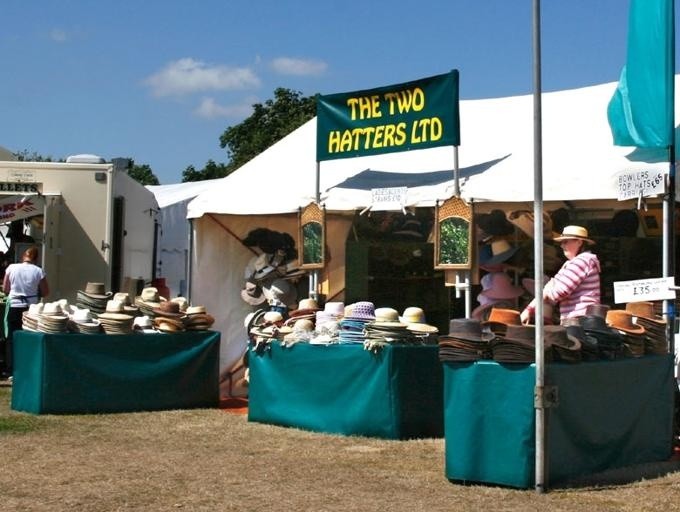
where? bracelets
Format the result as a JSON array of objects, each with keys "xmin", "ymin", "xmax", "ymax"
[{"xmin": 526, "ymin": 306, "xmax": 535, "ymax": 312}]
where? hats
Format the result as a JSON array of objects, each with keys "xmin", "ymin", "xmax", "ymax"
[
  {"xmin": 438, "ymin": 208, "xmax": 560, "ymax": 302},
  {"xmin": 553, "ymin": 225, "xmax": 597, "ymax": 245},
  {"xmin": 439, "ymin": 302, "xmax": 670, "ymax": 362},
  {"xmin": 22, "ymin": 280, "xmax": 217, "ymax": 337},
  {"xmin": 238, "ymin": 248, "xmax": 438, "ymax": 351}
]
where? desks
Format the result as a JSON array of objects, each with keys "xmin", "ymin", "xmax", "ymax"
[
  {"xmin": 244, "ymin": 331, "xmax": 444, "ymax": 440},
  {"xmin": 437, "ymin": 349, "xmax": 675, "ymax": 494},
  {"xmin": 9, "ymin": 328, "xmax": 223, "ymax": 414}
]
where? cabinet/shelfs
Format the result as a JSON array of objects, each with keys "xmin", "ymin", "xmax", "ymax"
[{"xmin": 341, "ymin": 238, "xmax": 451, "ymax": 337}]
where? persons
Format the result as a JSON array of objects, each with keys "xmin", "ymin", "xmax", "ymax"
[
  {"xmin": 0, "ymin": 247, "xmax": 48, "ymax": 377},
  {"xmin": 520, "ymin": 226, "xmax": 601, "ymax": 326}
]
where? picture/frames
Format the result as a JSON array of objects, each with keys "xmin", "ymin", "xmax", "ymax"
[
  {"xmin": 634, "ymin": 204, "xmax": 680, "ymax": 238},
  {"xmin": 430, "ymin": 193, "xmax": 475, "ymax": 271},
  {"xmin": 295, "ymin": 201, "xmax": 330, "ymax": 272}
]
